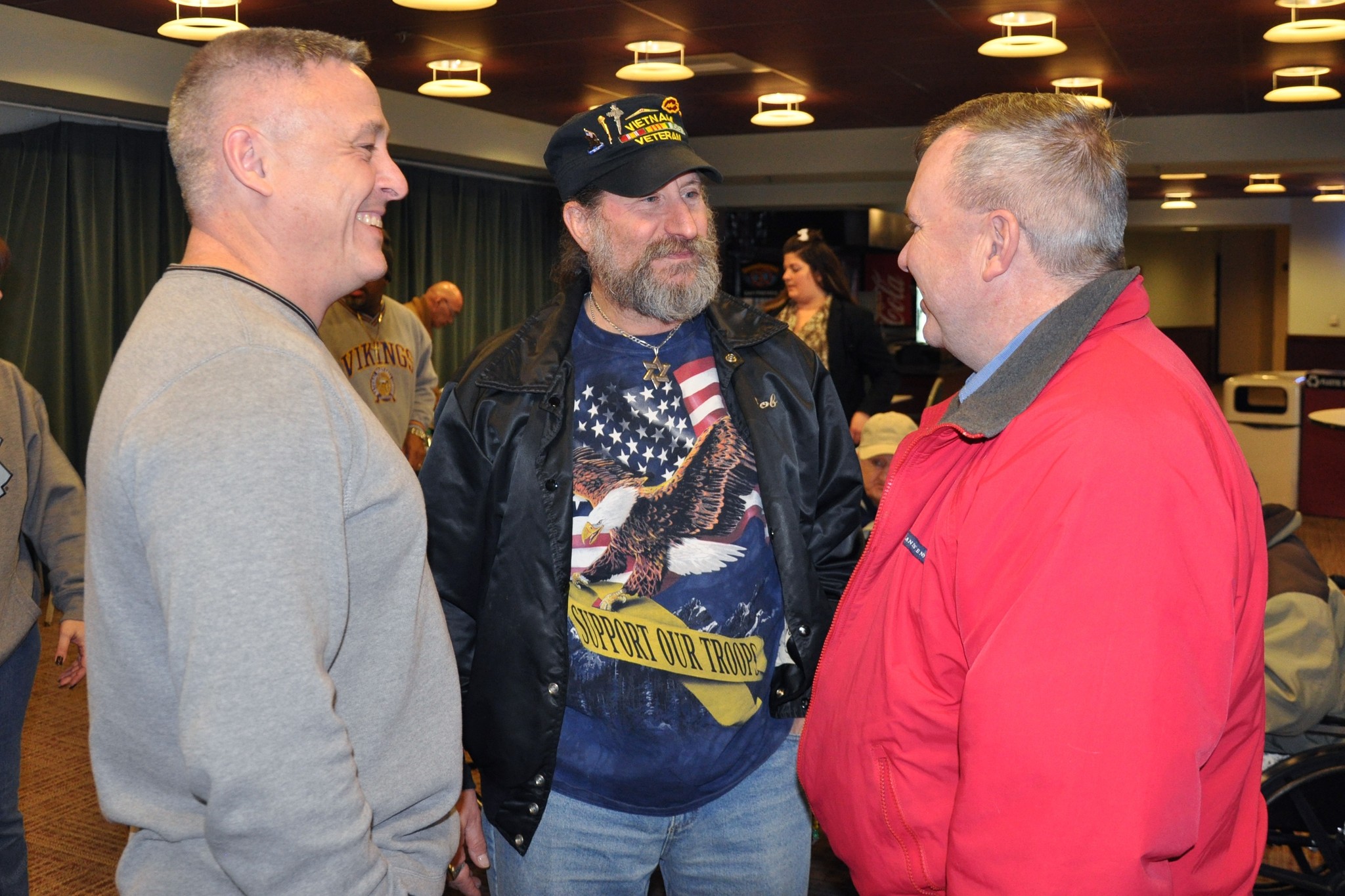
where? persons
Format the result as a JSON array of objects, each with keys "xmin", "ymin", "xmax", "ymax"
[
  {"xmin": 317, "ymin": 230, "xmax": 436, "ymax": 473},
  {"xmin": 0, "ymin": 361, "xmax": 89, "ymax": 896},
  {"xmin": 759, "ymin": 229, "xmax": 920, "ymax": 541},
  {"xmin": 403, "ymin": 281, "xmax": 462, "ymax": 403},
  {"xmin": 418, "ymin": 87, "xmax": 870, "ymax": 896},
  {"xmin": 796, "ymin": 88, "xmax": 1270, "ymax": 896},
  {"xmin": 83, "ymin": 25, "xmax": 463, "ymax": 895}
]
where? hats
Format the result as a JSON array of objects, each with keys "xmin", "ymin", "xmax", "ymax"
[
  {"xmin": 543, "ymin": 93, "xmax": 723, "ymax": 203},
  {"xmin": 855, "ymin": 411, "xmax": 919, "ymax": 460}
]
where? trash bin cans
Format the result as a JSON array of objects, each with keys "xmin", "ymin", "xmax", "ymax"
[{"xmin": 1222, "ymin": 371, "xmax": 1301, "ymax": 512}]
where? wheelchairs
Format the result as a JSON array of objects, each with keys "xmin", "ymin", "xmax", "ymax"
[{"xmin": 1254, "ymin": 715, "xmax": 1345, "ymax": 895}]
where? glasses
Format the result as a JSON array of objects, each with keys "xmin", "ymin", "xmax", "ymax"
[{"xmin": 444, "ymin": 298, "xmax": 458, "ymax": 319}]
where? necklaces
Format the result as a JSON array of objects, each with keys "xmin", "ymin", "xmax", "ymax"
[
  {"xmin": 588, "ymin": 295, "xmax": 596, "ymax": 327},
  {"xmin": 356, "ymin": 310, "xmax": 383, "ymax": 359},
  {"xmin": 591, "ymin": 292, "xmax": 684, "ymax": 389}
]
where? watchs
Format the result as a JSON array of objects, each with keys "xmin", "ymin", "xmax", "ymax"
[{"xmin": 408, "ymin": 428, "xmax": 432, "ymax": 449}]
